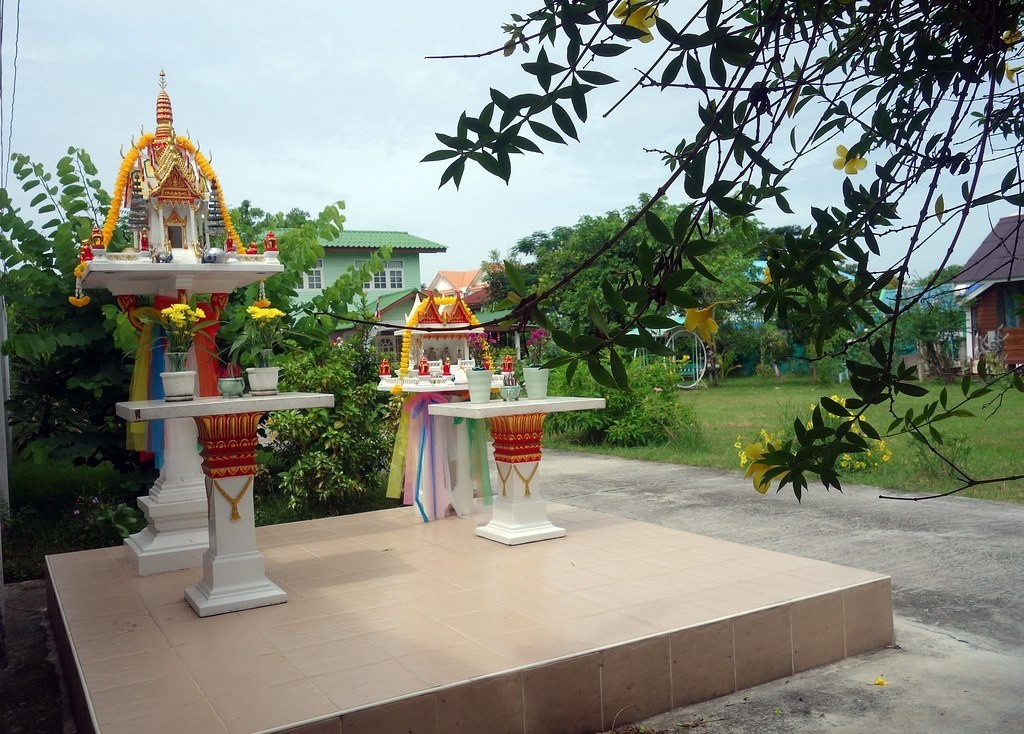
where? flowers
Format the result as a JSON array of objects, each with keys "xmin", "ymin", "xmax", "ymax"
[
  {"xmin": 66, "ymin": 132, "xmax": 285, "ymax": 325},
  {"xmin": 525, "ymin": 328, "xmax": 552, "ymax": 367},
  {"xmin": 120, "ymin": 304, "xmax": 222, "ymax": 368},
  {"xmin": 228, "ymin": 299, "xmax": 286, "ymax": 366},
  {"xmin": 389, "ymin": 295, "xmax": 496, "ymax": 389},
  {"xmin": 466, "ymin": 331, "xmax": 497, "ymax": 371}
]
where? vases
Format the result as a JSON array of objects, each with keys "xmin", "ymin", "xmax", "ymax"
[
  {"xmin": 523, "ymin": 368, "xmax": 549, "ymax": 400},
  {"xmin": 245, "ymin": 366, "xmax": 280, "ymax": 396},
  {"xmin": 159, "ymin": 370, "xmax": 197, "ymax": 402},
  {"xmin": 466, "ymin": 371, "xmax": 492, "ymax": 404}
]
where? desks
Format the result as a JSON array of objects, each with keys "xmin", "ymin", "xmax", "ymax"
[
  {"xmin": 115, "ymin": 392, "xmax": 334, "ymax": 618},
  {"xmin": 378, "ymin": 381, "xmax": 515, "ymax": 523},
  {"xmin": 427, "ymin": 396, "xmax": 606, "ymax": 546},
  {"xmin": 76, "ymin": 256, "xmax": 286, "ymax": 577}
]
